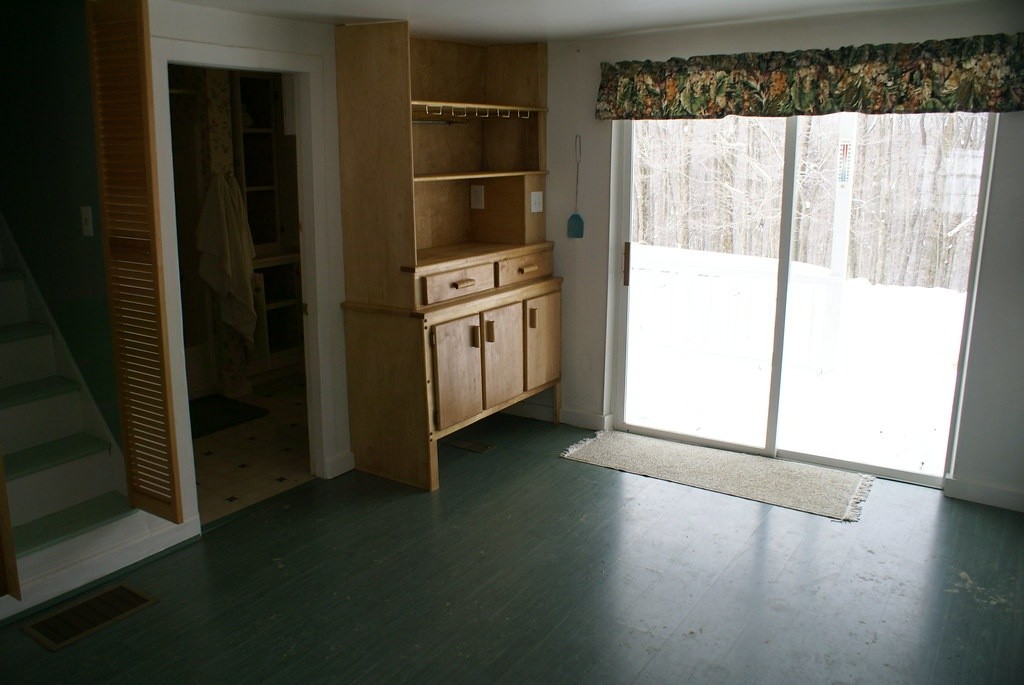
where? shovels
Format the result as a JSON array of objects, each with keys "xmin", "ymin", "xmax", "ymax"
[{"xmin": 567, "ymin": 135, "xmax": 584, "ymax": 238}]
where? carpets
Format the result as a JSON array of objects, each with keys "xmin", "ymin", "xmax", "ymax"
[{"xmin": 559, "ymin": 429, "xmax": 875, "ymax": 522}]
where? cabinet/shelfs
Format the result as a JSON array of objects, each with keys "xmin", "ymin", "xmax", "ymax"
[
  {"xmin": 331, "ymin": 19, "xmax": 565, "ymax": 490},
  {"xmin": 195, "ymin": 72, "xmax": 304, "ymax": 390}
]
